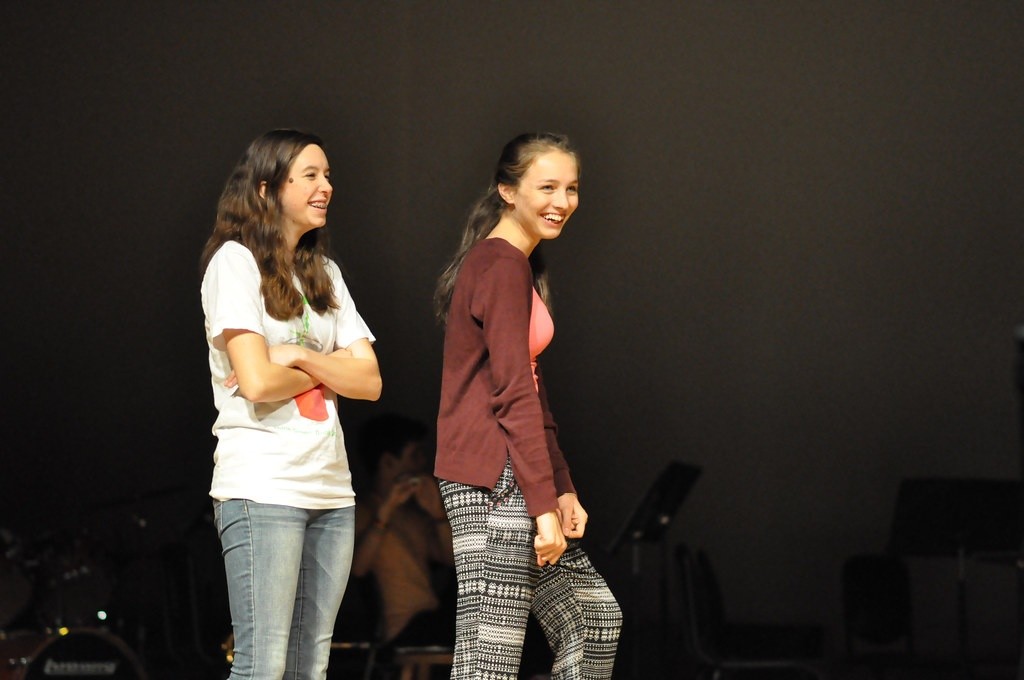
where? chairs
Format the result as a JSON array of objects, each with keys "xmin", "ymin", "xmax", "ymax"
[
  {"xmin": 675, "ymin": 541, "xmax": 827, "ymax": 680},
  {"xmin": 348, "ymin": 575, "xmax": 453, "ymax": 680},
  {"xmin": 841, "ymin": 553, "xmax": 963, "ymax": 679}
]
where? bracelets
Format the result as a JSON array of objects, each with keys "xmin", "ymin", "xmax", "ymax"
[{"xmin": 432, "ymin": 517, "xmax": 448, "ymax": 525}]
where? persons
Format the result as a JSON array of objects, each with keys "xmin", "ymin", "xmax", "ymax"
[
  {"xmin": 433, "ymin": 132, "xmax": 622, "ymax": 680},
  {"xmin": 351, "ymin": 414, "xmax": 457, "ymax": 648},
  {"xmin": 201, "ymin": 129, "xmax": 383, "ymax": 680}
]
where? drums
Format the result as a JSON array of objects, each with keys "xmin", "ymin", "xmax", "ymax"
[{"xmin": 0, "ymin": 623, "xmax": 146, "ymax": 680}]
